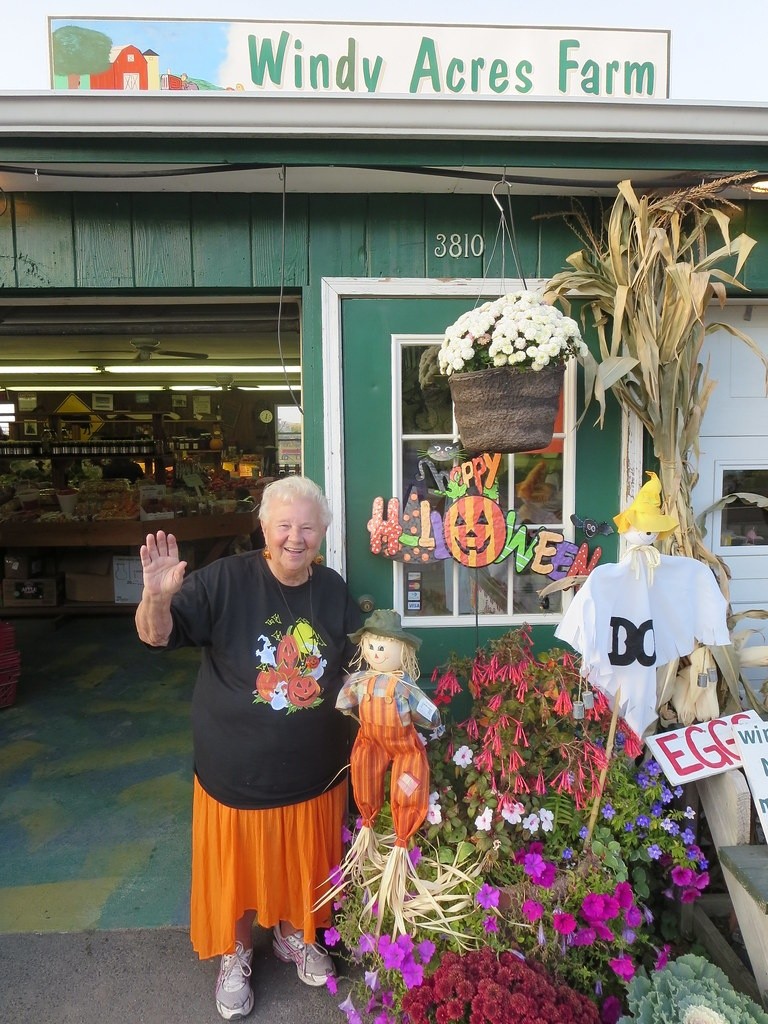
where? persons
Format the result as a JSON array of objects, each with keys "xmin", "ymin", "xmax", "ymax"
[
  {"xmin": 336, "ymin": 610, "xmax": 439, "ymax": 849},
  {"xmin": 134, "ymin": 475, "xmax": 364, "ymax": 1021}
]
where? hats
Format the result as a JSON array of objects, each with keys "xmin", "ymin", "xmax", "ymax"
[
  {"xmin": 346, "ymin": 609, "xmax": 422, "ymax": 651},
  {"xmin": 613, "ymin": 469, "xmax": 680, "ymax": 541}
]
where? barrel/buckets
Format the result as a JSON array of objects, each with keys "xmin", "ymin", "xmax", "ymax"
[
  {"xmin": 18, "ymin": 489, "xmax": 40, "ymax": 512},
  {"xmin": 57, "ymin": 489, "xmax": 77, "ymax": 513}
]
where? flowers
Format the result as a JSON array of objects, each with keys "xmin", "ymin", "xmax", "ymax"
[{"xmin": 437, "ymin": 289, "xmax": 590, "ymax": 376}]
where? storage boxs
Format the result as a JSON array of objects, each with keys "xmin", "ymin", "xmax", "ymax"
[{"xmin": 0, "ymin": 550, "xmax": 144, "ymax": 709}]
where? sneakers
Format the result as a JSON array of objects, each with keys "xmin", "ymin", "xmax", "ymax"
[
  {"xmin": 214, "ymin": 939, "xmax": 255, "ymax": 1021},
  {"xmin": 272, "ymin": 920, "xmax": 337, "ymax": 986}
]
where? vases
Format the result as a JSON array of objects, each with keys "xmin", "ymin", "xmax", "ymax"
[{"xmin": 447, "ymin": 364, "xmax": 566, "ymax": 453}]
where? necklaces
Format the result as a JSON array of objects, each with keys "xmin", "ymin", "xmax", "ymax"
[{"xmin": 274, "ymin": 568, "xmax": 319, "ymax": 669}]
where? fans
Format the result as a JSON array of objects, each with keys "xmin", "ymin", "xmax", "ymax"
[{"xmin": 78, "ymin": 337, "xmax": 209, "ymax": 363}]
